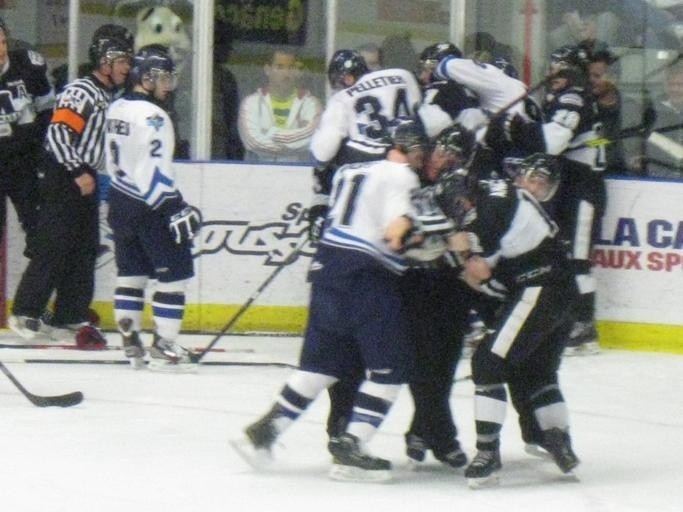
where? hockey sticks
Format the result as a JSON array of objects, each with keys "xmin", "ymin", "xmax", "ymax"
[
  {"xmin": 567, "ymin": 107, "xmax": 657, "ymax": 158},
  {"xmin": 490, "ymin": 66, "xmax": 578, "ymax": 113},
  {"xmin": 183, "ymin": 233, "xmax": 313, "ymax": 361},
  {"xmin": 0, "ymin": 366, "xmax": 83, "ymax": 407}
]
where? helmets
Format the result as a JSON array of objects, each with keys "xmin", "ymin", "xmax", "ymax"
[
  {"xmin": 327, "ymin": 49, "xmax": 366, "ymax": 89},
  {"xmin": 433, "ymin": 166, "xmax": 484, "ymax": 221},
  {"xmin": 550, "ymin": 47, "xmax": 586, "ymax": 74},
  {"xmin": 88, "ymin": 37, "xmax": 134, "ymax": 63},
  {"xmin": 381, "ymin": 116, "xmax": 432, "ymax": 154},
  {"xmin": 515, "ymin": 153, "xmax": 565, "ymax": 205},
  {"xmin": 128, "ymin": 44, "xmax": 179, "ymax": 81},
  {"xmin": 419, "ymin": 40, "xmax": 462, "ymax": 70},
  {"xmin": 491, "ymin": 55, "xmax": 519, "ymax": 81},
  {"xmin": 433, "ymin": 124, "xmax": 476, "ymax": 163}
]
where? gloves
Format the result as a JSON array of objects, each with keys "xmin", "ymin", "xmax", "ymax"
[
  {"xmin": 156, "ymin": 197, "xmax": 204, "ymax": 247},
  {"xmin": 313, "ymin": 168, "xmax": 335, "ymax": 192}
]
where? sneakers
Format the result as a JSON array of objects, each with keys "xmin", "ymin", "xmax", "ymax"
[
  {"xmin": 150, "ymin": 332, "xmax": 194, "ymax": 362},
  {"xmin": 463, "ymin": 448, "xmax": 503, "ymax": 480},
  {"xmin": 123, "ymin": 331, "xmax": 147, "ymax": 359},
  {"xmin": 566, "ymin": 324, "xmax": 599, "ymax": 347},
  {"xmin": 332, "ymin": 452, "xmax": 391, "ymax": 472},
  {"xmin": 435, "ymin": 437, "xmax": 467, "ymax": 469},
  {"xmin": 244, "ymin": 414, "xmax": 286, "ymax": 466},
  {"xmin": 53, "ymin": 313, "xmax": 89, "ymax": 331},
  {"xmin": 328, "ymin": 435, "xmax": 372, "ymax": 462},
  {"xmin": 405, "ymin": 432, "xmax": 429, "ymax": 462},
  {"xmin": 519, "ymin": 416, "xmax": 539, "ymax": 446},
  {"xmin": 10, "ymin": 308, "xmax": 42, "ymax": 334},
  {"xmin": 550, "ymin": 438, "xmax": 580, "ymax": 474}
]
